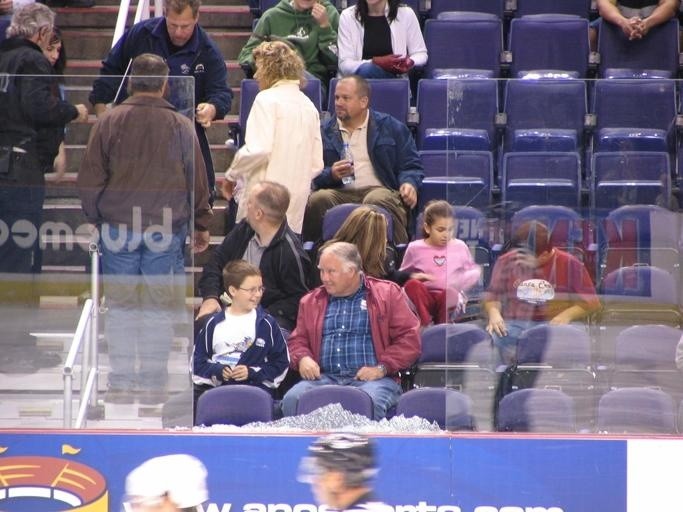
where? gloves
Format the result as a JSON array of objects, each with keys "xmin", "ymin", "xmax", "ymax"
[
  {"xmin": 372, "ymin": 55, "xmax": 401, "ymax": 70},
  {"xmin": 392, "ymin": 56, "xmax": 414, "ymax": 73}
]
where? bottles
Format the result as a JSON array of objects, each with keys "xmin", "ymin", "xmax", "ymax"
[{"xmin": 338, "ymin": 141, "xmax": 356, "ymax": 185}]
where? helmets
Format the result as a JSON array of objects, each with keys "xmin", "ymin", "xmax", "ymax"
[{"xmin": 295, "ymin": 435, "xmax": 378, "ymax": 489}]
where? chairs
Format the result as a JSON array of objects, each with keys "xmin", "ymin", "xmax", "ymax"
[{"xmin": 189, "ymin": 0, "xmax": 682, "ymax": 434}]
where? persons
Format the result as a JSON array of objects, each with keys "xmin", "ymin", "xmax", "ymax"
[
  {"xmin": 588, "ymin": 0, "xmax": 683, "ymax": 73},
  {"xmin": 297, "ymin": 429, "xmax": 386, "ymax": 511}
]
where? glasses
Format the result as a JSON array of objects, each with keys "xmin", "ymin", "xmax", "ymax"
[{"xmin": 237, "ymin": 287, "xmax": 266, "ymax": 294}]
